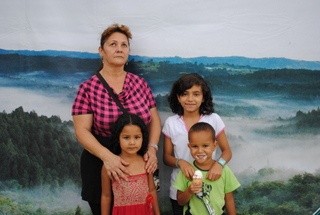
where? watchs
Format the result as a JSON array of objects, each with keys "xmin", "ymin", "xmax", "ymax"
[{"xmin": 147, "ymin": 144, "xmax": 158, "ymax": 150}]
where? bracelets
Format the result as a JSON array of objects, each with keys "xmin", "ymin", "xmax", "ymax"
[
  {"xmin": 176, "ymin": 159, "xmax": 178, "ymax": 168},
  {"xmin": 217, "ymin": 158, "xmax": 227, "ymax": 167}
]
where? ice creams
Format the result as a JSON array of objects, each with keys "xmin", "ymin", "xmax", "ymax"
[{"xmin": 193, "ymin": 170, "xmax": 203, "ymax": 196}]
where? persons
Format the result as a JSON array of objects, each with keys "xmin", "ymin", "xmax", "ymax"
[
  {"xmin": 71, "ymin": 23, "xmax": 162, "ymax": 215},
  {"xmin": 173, "ymin": 122, "xmax": 241, "ymax": 215},
  {"xmin": 162, "ymin": 72, "xmax": 232, "ymax": 215},
  {"xmin": 101, "ymin": 114, "xmax": 160, "ymax": 215}
]
